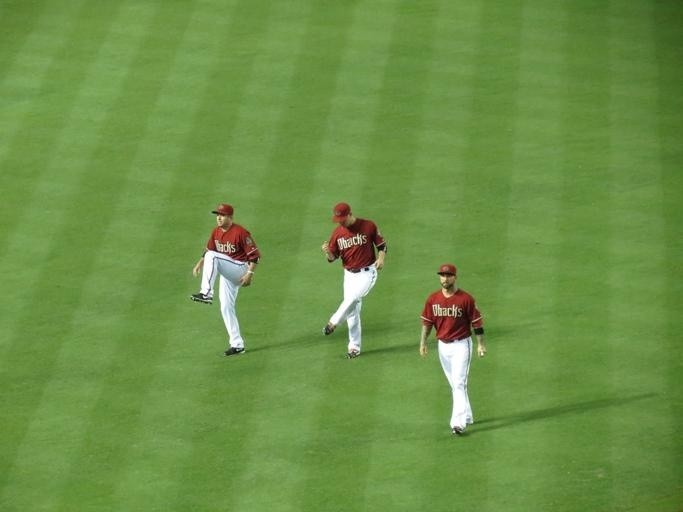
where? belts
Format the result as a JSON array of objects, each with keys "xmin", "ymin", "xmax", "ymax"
[
  {"xmin": 348, "ymin": 267, "xmax": 368, "ymax": 273},
  {"xmin": 440, "ymin": 334, "xmax": 473, "ymax": 343}
]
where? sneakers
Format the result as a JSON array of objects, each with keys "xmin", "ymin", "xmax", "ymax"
[
  {"xmin": 190, "ymin": 293, "xmax": 213, "ymax": 304},
  {"xmin": 224, "ymin": 347, "xmax": 245, "ymax": 358},
  {"xmin": 322, "ymin": 323, "xmax": 336, "ymax": 336},
  {"xmin": 452, "ymin": 426, "xmax": 466, "ymax": 435},
  {"xmin": 344, "ymin": 350, "xmax": 361, "ymax": 359}
]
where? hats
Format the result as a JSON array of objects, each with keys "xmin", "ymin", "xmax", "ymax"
[
  {"xmin": 437, "ymin": 265, "xmax": 457, "ymax": 274},
  {"xmin": 212, "ymin": 204, "xmax": 234, "ymax": 215},
  {"xmin": 332, "ymin": 203, "xmax": 351, "ymax": 222}
]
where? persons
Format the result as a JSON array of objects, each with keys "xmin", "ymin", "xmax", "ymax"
[
  {"xmin": 187, "ymin": 204, "xmax": 261, "ymax": 355},
  {"xmin": 321, "ymin": 202, "xmax": 388, "ymax": 361},
  {"xmin": 419, "ymin": 264, "xmax": 487, "ymax": 434}
]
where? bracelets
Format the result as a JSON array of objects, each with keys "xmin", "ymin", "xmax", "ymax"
[{"xmin": 246, "ymin": 270, "xmax": 255, "ymax": 276}]
